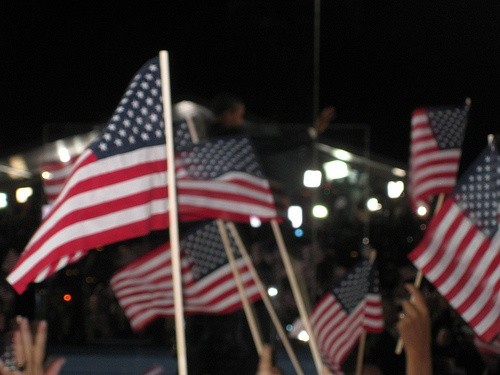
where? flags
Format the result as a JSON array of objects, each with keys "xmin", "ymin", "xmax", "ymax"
[{"xmin": 5, "ymin": 49, "xmax": 179, "ymax": 294}]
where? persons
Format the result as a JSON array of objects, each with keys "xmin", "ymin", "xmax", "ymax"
[{"xmin": 0, "ymin": 100, "xmax": 500, "ymax": 375}]
